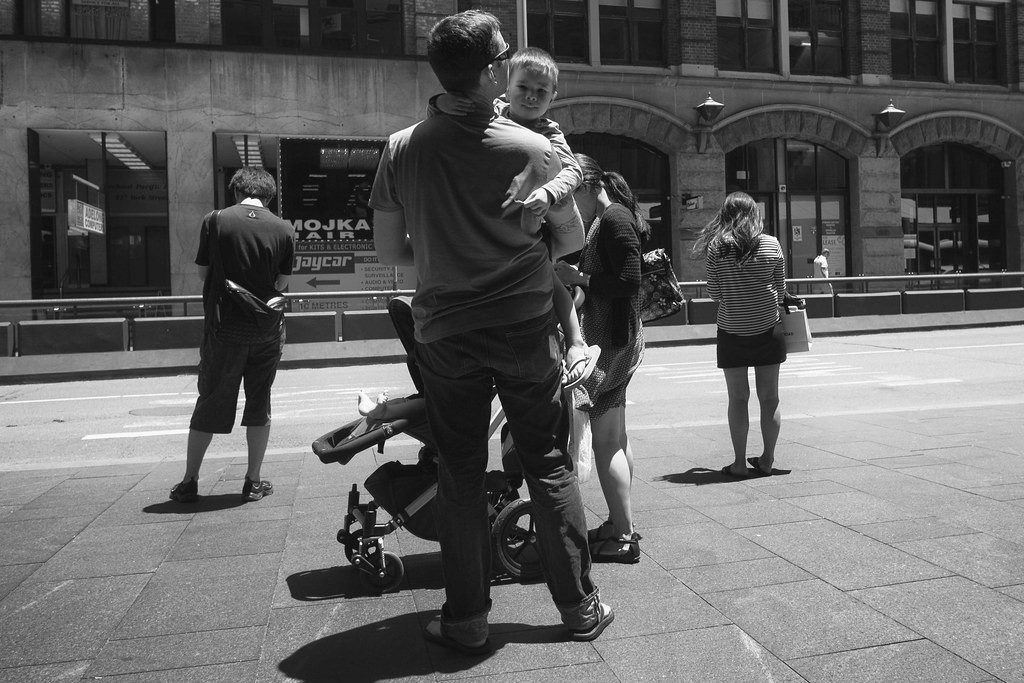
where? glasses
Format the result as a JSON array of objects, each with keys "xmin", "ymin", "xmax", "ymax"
[{"xmin": 482, "ymin": 41, "xmax": 512, "ymax": 68}]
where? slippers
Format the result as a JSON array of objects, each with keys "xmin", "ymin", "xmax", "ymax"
[
  {"xmin": 424, "ymin": 614, "xmax": 491, "ymax": 656},
  {"xmin": 571, "ymin": 602, "xmax": 613, "ymax": 641},
  {"xmin": 722, "ymin": 463, "xmax": 751, "ymax": 478},
  {"xmin": 747, "ymin": 457, "xmax": 773, "ymax": 476},
  {"xmin": 562, "ymin": 344, "xmax": 601, "ymax": 390}
]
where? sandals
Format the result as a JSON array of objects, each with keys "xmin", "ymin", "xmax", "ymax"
[
  {"xmin": 589, "ymin": 532, "xmax": 640, "ymax": 561},
  {"xmin": 587, "ymin": 521, "xmax": 633, "ymax": 541}
]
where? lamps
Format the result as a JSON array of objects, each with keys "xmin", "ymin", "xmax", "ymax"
[
  {"xmin": 872, "ymin": 98, "xmax": 906, "ymax": 127},
  {"xmin": 694, "ymin": 90, "xmax": 726, "ymax": 122}
]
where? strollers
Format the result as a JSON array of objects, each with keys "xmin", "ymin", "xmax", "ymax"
[{"xmin": 312, "ymin": 285, "xmax": 587, "ymax": 594}]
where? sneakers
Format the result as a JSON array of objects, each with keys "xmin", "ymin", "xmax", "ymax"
[
  {"xmin": 242, "ymin": 475, "xmax": 273, "ymax": 500},
  {"xmin": 169, "ymin": 477, "xmax": 198, "ymax": 508}
]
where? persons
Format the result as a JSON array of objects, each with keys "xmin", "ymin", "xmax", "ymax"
[
  {"xmin": 367, "ymin": 12, "xmax": 616, "ymax": 655},
  {"xmin": 424, "ymin": 45, "xmax": 604, "ymax": 391},
  {"xmin": 168, "ymin": 165, "xmax": 298, "ymax": 505},
  {"xmin": 706, "ymin": 191, "xmax": 787, "ymax": 480},
  {"xmin": 551, "ymin": 153, "xmax": 646, "ymax": 564},
  {"xmin": 812, "ymin": 248, "xmax": 834, "ymax": 294},
  {"xmin": 357, "ymin": 389, "xmax": 427, "ymax": 421}
]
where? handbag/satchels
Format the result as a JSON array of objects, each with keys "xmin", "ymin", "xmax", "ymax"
[
  {"xmin": 639, "ymin": 247, "xmax": 686, "ymax": 323},
  {"xmin": 778, "ymin": 289, "xmax": 807, "ymax": 313},
  {"xmin": 212, "ymin": 277, "xmax": 287, "ymax": 346},
  {"xmin": 780, "ymin": 295, "xmax": 813, "ymax": 353}
]
